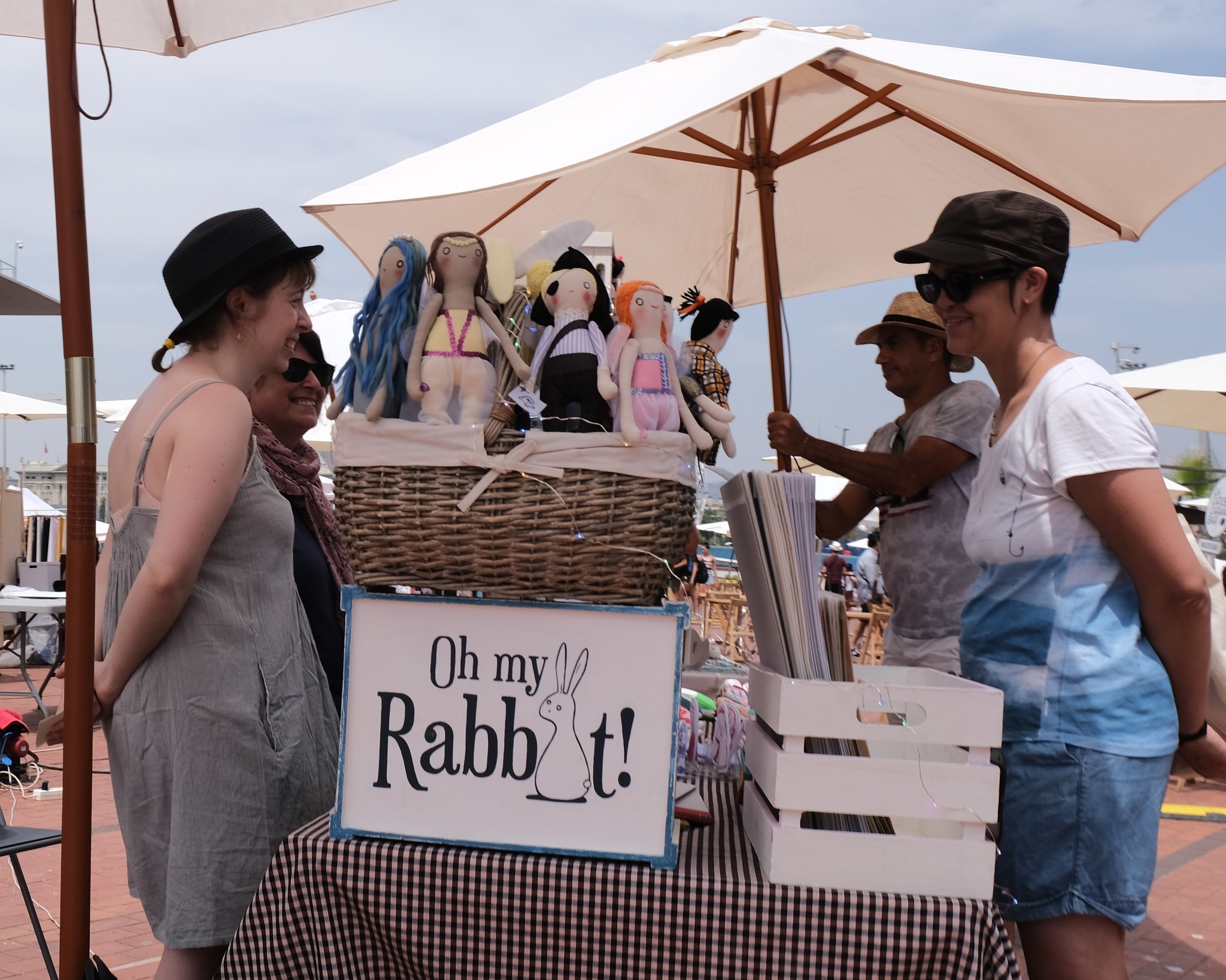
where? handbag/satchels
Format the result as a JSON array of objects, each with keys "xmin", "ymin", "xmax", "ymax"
[{"xmin": 696, "ymin": 559, "xmax": 708, "ymax": 583}]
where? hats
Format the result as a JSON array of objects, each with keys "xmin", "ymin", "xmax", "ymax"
[
  {"xmin": 829, "ymin": 541, "xmax": 843, "ymax": 552},
  {"xmin": 894, "ymin": 189, "xmax": 1069, "ymax": 286},
  {"xmin": 856, "ymin": 292, "xmax": 974, "ymax": 372},
  {"xmin": 163, "ymin": 208, "xmax": 324, "ymax": 331}
]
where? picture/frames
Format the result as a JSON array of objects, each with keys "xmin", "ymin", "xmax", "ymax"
[{"xmin": 324, "ymin": 581, "xmax": 687, "ymax": 874}]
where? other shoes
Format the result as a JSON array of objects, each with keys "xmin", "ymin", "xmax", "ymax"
[{"xmin": 851, "ymin": 647, "xmax": 861, "ymax": 659}]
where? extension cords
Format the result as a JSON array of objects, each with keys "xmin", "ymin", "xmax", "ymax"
[{"xmin": 32, "ymin": 787, "xmax": 63, "ymax": 801}]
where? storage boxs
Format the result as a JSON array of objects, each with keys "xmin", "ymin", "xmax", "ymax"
[{"xmin": 738, "ymin": 659, "xmax": 1009, "ymax": 908}]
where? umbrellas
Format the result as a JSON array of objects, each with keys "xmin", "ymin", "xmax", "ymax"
[
  {"xmin": 0, "ymin": 0, "xmax": 374, "ymax": 980},
  {"xmin": 1110, "ymin": 353, "xmax": 1226, "ymax": 433},
  {"xmin": 300, "ymin": 17, "xmax": 1226, "ymax": 477}
]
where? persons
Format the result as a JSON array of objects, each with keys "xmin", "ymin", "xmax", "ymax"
[
  {"xmin": 45, "ymin": 210, "xmax": 340, "ymax": 980},
  {"xmin": 674, "ymin": 526, "xmax": 720, "ymax": 620},
  {"xmin": 768, "ymin": 292, "xmax": 999, "ymax": 675},
  {"xmin": 849, "ymin": 531, "xmax": 885, "ymax": 652},
  {"xmin": 894, "ymin": 188, "xmax": 1226, "ymax": 980},
  {"xmin": 247, "ymin": 330, "xmax": 355, "ymax": 719},
  {"xmin": 819, "ymin": 540, "xmax": 861, "ymax": 604}
]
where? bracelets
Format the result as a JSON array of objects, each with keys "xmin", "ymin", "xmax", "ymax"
[
  {"xmin": 801, "ymin": 433, "xmax": 810, "ymax": 451},
  {"xmin": 1176, "ymin": 722, "xmax": 1210, "ymax": 744}
]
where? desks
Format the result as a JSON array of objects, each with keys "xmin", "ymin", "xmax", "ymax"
[
  {"xmin": 0, "ymin": 579, "xmax": 67, "ymax": 726},
  {"xmin": 280, "ymin": 761, "xmax": 1013, "ymax": 980}
]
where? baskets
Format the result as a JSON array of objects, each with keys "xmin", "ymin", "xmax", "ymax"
[{"xmin": 328, "ymin": 288, "xmax": 697, "ymax": 607}]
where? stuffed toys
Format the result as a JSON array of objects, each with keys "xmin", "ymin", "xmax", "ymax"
[{"xmin": 325, "ymin": 230, "xmax": 739, "ymax": 467}]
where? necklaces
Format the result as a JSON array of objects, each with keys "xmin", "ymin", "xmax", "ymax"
[{"xmin": 988, "ymin": 339, "xmax": 1059, "ymax": 437}]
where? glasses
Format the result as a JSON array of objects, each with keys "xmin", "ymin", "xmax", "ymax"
[
  {"xmin": 283, "ymin": 358, "xmax": 335, "ymax": 388},
  {"xmin": 914, "ymin": 266, "xmax": 1018, "ymax": 303}
]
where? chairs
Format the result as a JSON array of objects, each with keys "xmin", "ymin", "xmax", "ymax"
[
  {"xmin": 700, "ymin": 585, "xmax": 890, "ymax": 664},
  {"xmin": 0, "ymin": 803, "xmax": 63, "ymax": 980}
]
